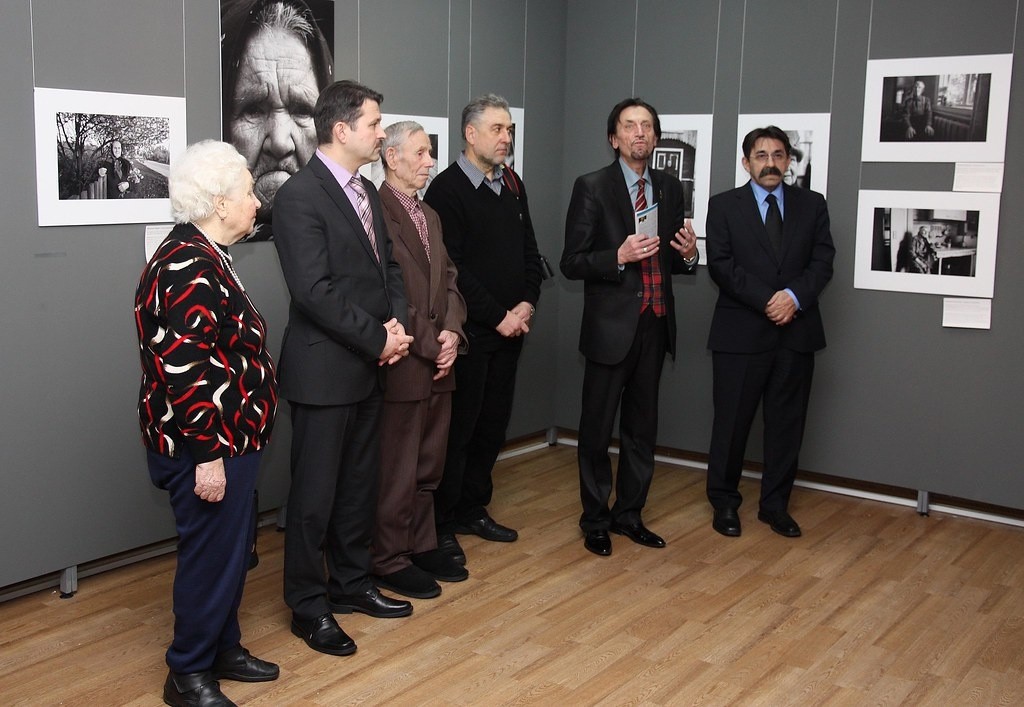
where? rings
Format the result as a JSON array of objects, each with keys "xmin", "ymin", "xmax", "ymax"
[
  {"xmin": 684, "ymin": 242, "xmax": 689, "ymax": 247},
  {"xmin": 644, "ymin": 247, "xmax": 648, "ymax": 252}
]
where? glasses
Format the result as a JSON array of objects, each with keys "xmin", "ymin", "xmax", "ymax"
[{"xmin": 749, "ymin": 152, "xmax": 789, "ymax": 162}]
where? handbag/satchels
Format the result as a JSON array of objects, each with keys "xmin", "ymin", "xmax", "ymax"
[{"xmin": 249, "ymin": 488, "xmax": 260, "ymax": 570}]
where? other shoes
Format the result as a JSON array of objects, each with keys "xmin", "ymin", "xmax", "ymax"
[
  {"xmin": 406, "ymin": 548, "xmax": 469, "ymax": 582},
  {"xmin": 374, "ymin": 564, "xmax": 442, "ymax": 599}
]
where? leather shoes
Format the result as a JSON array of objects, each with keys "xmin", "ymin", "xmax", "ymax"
[
  {"xmin": 607, "ymin": 519, "xmax": 666, "ymax": 548},
  {"xmin": 580, "ymin": 527, "xmax": 612, "ymax": 556},
  {"xmin": 456, "ymin": 514, "xmax": 518, "ymax": 542},
  {"xmin": 758, "ymin": 510, "xmax": 801, "ymax": 537},
  {"xmin": 327, "ymin": 584, "xmax": 414, "ymax": 618},
  {"xmin": 211, "ymin": 645, "xmax": 280, "ymax": 682},
  {"xmin": 162, "ymin": 669, "xmax": 238, "ymax": 707},
  {"xmin": 291, "ymin": 609, "xmax": 357, "ymax": 656},
  {"xmin": 713, "ymin": 506, "xmax": 741, "ymax": 537},
  {"xmin": 438, "ymin": 535, "xmax": 467, "ymax": 567}
]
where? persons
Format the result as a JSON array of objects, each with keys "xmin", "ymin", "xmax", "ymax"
[
  {"xmin": 908, "ymin": 226, "xmax": 937, "ymax": 274},
  {"xmin": 423, "ymin": 93, "xmax": 543, "ymax": 567},
  {"xmin": 705, "ymin": 125, "xmax": 836, "ymax": 536},
  {"xmin": 135, "ymin": 138, "xmax": 279, "ymax": 707},
  {"xmin": 782, "ymin": 130, "xmax": 804, "ymax": 186},
  {"xmin": 97, "ymin": 139, "xmax": 135, "ymax": 199},
  {"xmin": 271, "ymin": 79, "xmax": 414, "ymax": 656},
  {"xmin": 903, "ymin": 83, "xmax": 935, "ymax": 141},
  {"xmin": 377, "ymin": 121, "xmax": 469, "ymax": 599},
  {"xmin": 559, "ymin": 98, "xmax": 700, "ymax": 555},
  {"xmin": 221, "ymin": 0, "xmax": 333, "ymax": 240}
]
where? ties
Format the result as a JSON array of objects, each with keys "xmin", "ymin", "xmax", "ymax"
[
  {"xmin": 347, "ymin": 176, "xmax": 382, "ymax": 267},
  {"xmin": 635, "ymin": 178, "xmax": 648, "ymax": 212},
  {"xmin": 764, "ymin": 194, "xmax": 783, "ymax": 261}
]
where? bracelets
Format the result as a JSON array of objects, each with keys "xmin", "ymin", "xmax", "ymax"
[{"xmin": 529, "ymin": 303, "xmax": 535, "ymax": 316}]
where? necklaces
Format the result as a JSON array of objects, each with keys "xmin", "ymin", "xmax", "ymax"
[{"xmin": 191, "ymin": 221, "xmax": 246, "ymax": 292}]
